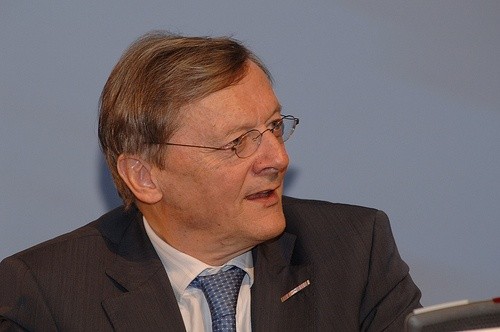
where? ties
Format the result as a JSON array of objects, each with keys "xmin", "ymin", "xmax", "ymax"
[{"xmin": 189, "ymin": 265, "xmax": 247, "ymax": 332}]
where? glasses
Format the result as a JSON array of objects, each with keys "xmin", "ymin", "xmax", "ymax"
[{"xmin": 136, "ymin": 114, "xmax": 299, "ymax": 158}]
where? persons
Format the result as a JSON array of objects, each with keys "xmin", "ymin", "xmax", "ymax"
[{"xmin": 0, "ymin": 31, "xmax": 424, "ymax": 332}]
